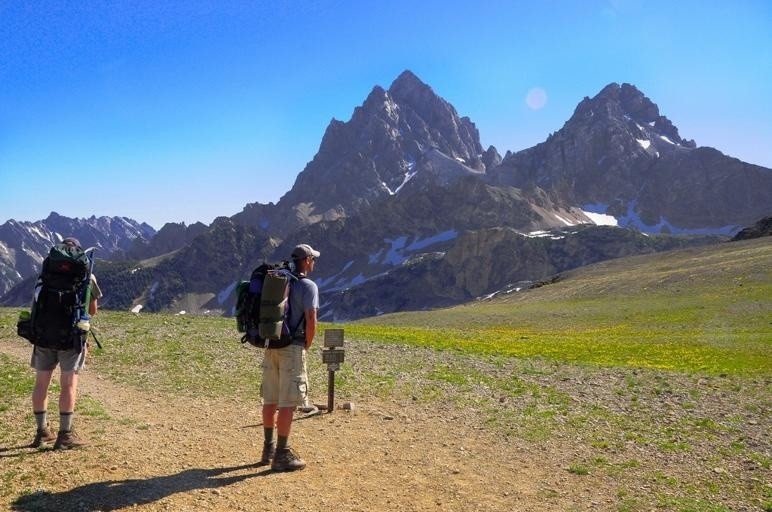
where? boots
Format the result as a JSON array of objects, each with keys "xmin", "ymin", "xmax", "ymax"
[
  {"xmin": 54, "ymin": 430, "xmax": 91, "ymax": 449},
  {"xmin": 33, "ymin": 426, "xmax": 56, "ymax": 446},
  {"xmin": 261, "ymin": 441, "xmax": 275, "ymax": 464},
  {"xmin": 271, "ymin": 449, "xmax": 306, "ymax": 471}
]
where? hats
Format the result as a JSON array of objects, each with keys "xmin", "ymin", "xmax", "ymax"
[
  {"xmin": 61, "ymin": 238, "xmax": 80, "ymax": 246},
  {"xmin": 291, "ymin": 244, "xmax": 320, "ymax": 260}
]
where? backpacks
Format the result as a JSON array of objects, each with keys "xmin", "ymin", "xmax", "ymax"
[
  {"xmin": 16, "ymin": 245, "xmax": 90, "ymax": 348},
  {"xmin": 235, "ymin": 260, "xmax": 307, "ymax": 348}
]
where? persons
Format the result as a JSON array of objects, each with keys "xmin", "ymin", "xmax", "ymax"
[
  {"xmin": 30, "ymin": 236, "xmax": 105, "ymax": 450},
  {"xmin": 258, "ymin": 243, "xmax": 321, "ymax": 472}
]
